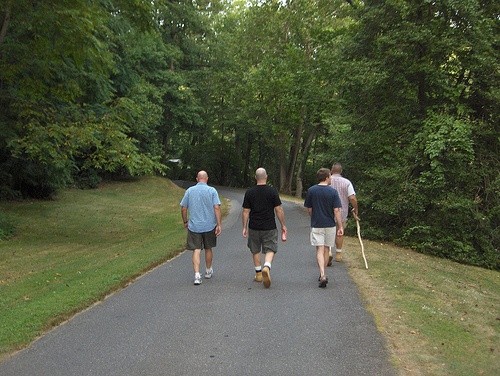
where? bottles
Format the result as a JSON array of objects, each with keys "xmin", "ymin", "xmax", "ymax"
[{"xmin": 282, "ymin": 228, "xmax": 286, "ymax": 242}]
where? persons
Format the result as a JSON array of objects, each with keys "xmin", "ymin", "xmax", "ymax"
[
  {"xmin": 303, "ymin": 168, "xmax": 344, "ymax": 288},
  {"xmin": 328, "ymin": 163, "xmax": 359, "ymax": 265},
  {"xmin": 179, "ymin": 170, "xmax": 221, "ymax": 284},
  {"xmin": 242, "ymin": 167, "xmax": 287, "ymax": 287}
]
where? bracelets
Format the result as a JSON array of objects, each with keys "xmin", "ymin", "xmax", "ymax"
[{"xmin": 184, "ymin": 221, "xmax": 188, "ymax": 223}]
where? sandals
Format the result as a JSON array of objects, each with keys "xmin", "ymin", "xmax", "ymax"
[{"xmin": 318, "ymin": 275, "xmax": 327, "ymax": 288}]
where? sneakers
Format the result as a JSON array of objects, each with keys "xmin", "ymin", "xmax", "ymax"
[
  {"xmin": 204, "ymin": 270, "xmax": 213, "ymax": 278},
  {"xmin": 194, "ymin": 276, "xmax": 202, "ymax": 284}
]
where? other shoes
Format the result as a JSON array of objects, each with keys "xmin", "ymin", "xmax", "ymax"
[
  {"xmin": 335, "ymin": 252, "xmax": 342, "ymax": 262},
  {"xmin": 255, "ymin": 269, "xmax": 262, "ymax": 282},
  {"xmin": 262, "ymin": 266, "xmax": 271, "ymax": 287},
  {"xmin": 327, "ymin": 253, "xmax": 333, "ymax": 266}
]
